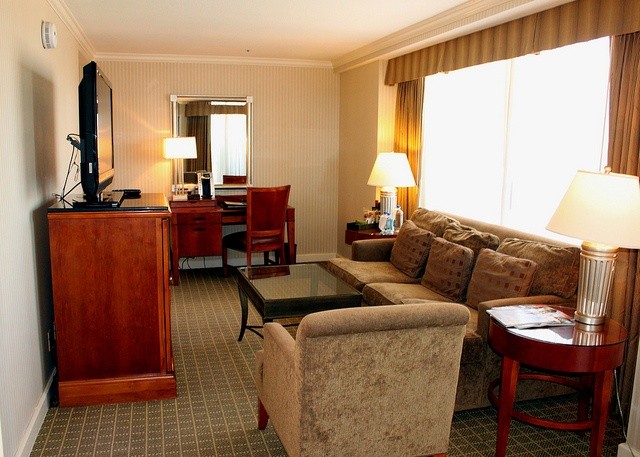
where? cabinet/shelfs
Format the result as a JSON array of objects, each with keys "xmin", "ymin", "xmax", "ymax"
[{"xmin": 48, "ymin": 193, "xmax": 177, "ymax": 411}]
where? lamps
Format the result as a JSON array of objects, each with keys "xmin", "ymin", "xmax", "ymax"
[
  {"xmin": 164, "ymin": 135, "xmax": 198, "ymax": 200},
  {"xmin": 367, "ymin": 150, "xmax": 415, "ymax": 223},
  {"xmin": 546, "ymin": 165, "xmax": 640, "ymax": 326}
]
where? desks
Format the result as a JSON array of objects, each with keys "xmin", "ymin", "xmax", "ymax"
[
  {"xmin": 485, "ymin": 302, "xmax": 629, "ymax": 456},
  {"xmin": 345, "ymin": 222, "xmax": 405, "ymax": 244},
  {"xmin": 169, "ymin": 200, "xmax": 295, "ymax": 286}
]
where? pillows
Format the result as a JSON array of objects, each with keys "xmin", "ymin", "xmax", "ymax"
[
  {"xmin": 421, "ymin": 236, "xmax": 475, "ymax": 301},
  {"xmin": 408, "ymin": 205, "xmax": 461, "ymax": 238},
  {"xmin": 496, "ymin": 236, "xmax": 578, "ymax": 298},
  {"xmin": 439, "ymin": 221, "xmax": 501, "ymax": 255},
  {"xmin": 390, "ymin": 219, "xmax": 437, "ymax": 278},
  {"xmin": 465, "ymin": 246, "xmax": 538, "ymax": 310}
]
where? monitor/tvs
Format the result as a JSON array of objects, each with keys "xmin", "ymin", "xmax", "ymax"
[{"xmin": 75, "ymin": 59, "xmax": 125, "ymax": 205}]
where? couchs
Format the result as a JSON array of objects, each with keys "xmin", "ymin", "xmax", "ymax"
[
  {"xmin": 324, "ymin": 205, "xmax": 617, "ymax": 413},
  {"xmin": 253, "ymin": 302, "xmax": 470, "ymax": 457}
]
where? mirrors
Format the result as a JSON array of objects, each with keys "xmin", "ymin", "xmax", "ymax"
[{"xmin": 169, "ymin": 93, "xmax": 253, "ymax": 200}]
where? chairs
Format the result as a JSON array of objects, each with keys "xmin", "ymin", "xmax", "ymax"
[{"xmin": 218, "ymin": 184, "xmax": 292, "ymax": 280}]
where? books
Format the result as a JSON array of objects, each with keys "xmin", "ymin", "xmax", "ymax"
[{"xmin": 485, "ymin": 304, "xmax": 575, "ymax": 330}]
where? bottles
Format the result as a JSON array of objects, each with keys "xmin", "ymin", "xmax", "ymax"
[
  {"xmin": 395, "ymin": 205, "xmax": 403, "ymax": 229},
  {"xmin": 379, "ymin": 211, "xmax": 387, "ymax": 231},
  {"xmin": 386, "ymin": 212, "xmax": 393, "ymax": 231}
]
